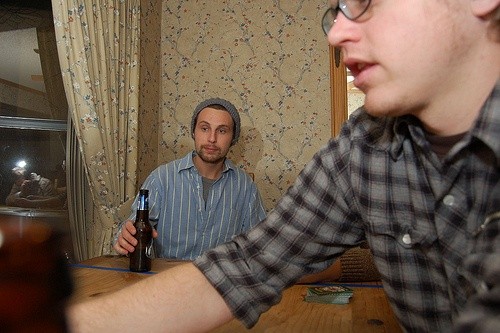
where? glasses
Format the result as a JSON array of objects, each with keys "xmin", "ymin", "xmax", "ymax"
[{"xmin": 321, "ymin": 0, "xmax": 372, "ymax": 36}]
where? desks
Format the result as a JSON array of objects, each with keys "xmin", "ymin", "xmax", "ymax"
[{"xmin": 69, "ymin": 255, "xmax": 407, "ymax": 333}]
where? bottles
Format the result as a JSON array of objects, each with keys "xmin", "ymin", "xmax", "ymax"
[{"xmin": 130, "ymin": 189, "xmax": 153, "ymax": 273}]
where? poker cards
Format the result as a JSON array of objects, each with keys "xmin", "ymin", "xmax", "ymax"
[{"xmin": 303, "ymin": 285, "xmax": 355, "ymax": 304}]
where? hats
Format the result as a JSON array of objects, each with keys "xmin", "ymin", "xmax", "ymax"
[{"xmin": 191, "ymin": 97, "xmax": 241, "ymax": 145}]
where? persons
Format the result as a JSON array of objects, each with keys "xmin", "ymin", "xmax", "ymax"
[
  {"xmin": 112, "ymin": 97, "xmax": 267, "ymax": 261},
  {"xmin": 0, "ymin": 0, "xmax": 500, "ymax": 333},
  {"xmin": 7, "ymin": 156, "xmax": 65, "ymax": 211},
  {"xmin": 0, "ymin": 217, "xmax": 73, "ymax": 333}
]
